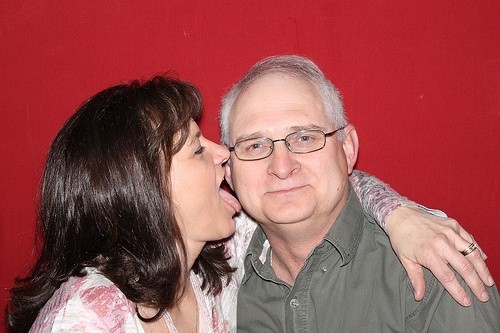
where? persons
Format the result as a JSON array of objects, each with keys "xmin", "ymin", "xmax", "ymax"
[
  {"xmin": 219, "ymin": 54, "xmax": 500, "ymax": 333},
  {"xmin": 4, "ymin": 71, "xmax": 496, "ymax": 333}
]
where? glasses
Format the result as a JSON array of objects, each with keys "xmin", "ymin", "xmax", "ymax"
[{"xmin": 228, "ymin": 125, "xmax": 345, "ymax": 162}]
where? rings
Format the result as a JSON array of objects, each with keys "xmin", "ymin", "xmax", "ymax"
[{"xmin": 461, "ymin": 242, "xmax": 477, "ymax": 256}]
